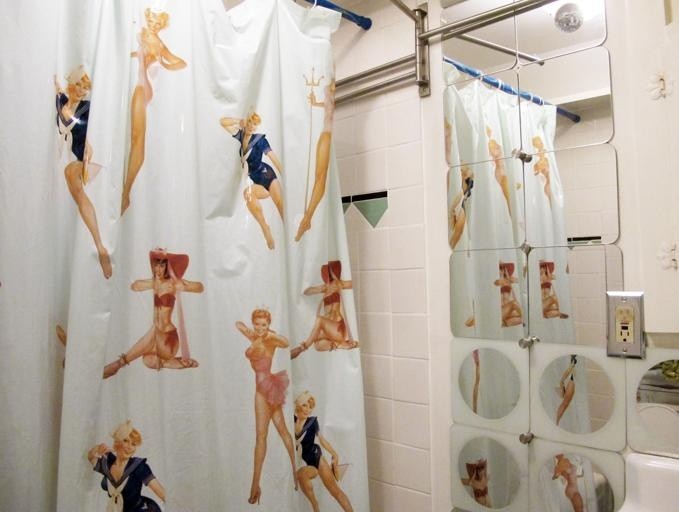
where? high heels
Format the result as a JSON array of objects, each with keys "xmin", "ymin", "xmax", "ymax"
[{"xmin": 248, "ymin": 488, "xmax": 261, "ymax": 505}]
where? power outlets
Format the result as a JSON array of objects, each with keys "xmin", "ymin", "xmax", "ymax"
[{"xmin": 606, "ymin": 292, "xmax": 645, "ymax": 360}]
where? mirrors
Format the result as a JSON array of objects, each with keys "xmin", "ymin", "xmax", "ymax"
[{"xmin": 441, "ymin": 0, "xmax": 628, "ymax": 512}]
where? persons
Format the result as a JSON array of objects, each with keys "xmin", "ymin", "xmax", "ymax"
[
  {"xmin": 235, "ymin": 308, "xmax": 297, "ymax": 505},
  {"xmin": 52, "ymin": 64, "xmax": 113, "ymax": 281},
  {"xmin": 294, "ymin": 390, "xmax": 354, "ymax": 512},
  {"xmin": 120, "ymin": 7, "xmax": 188, "ymax": 218},
  {"xmin": 290, "ymin": 258, "xmax": 359, "ymax": 359},
  {"xmin": 293, "ymin": 60, "xmax": 336, "ymax": 243},
  {"xmin": 102, "ymin": 245, "xmax": 205, "ymax": 380},
  {"xmin": 87, "ymin": 420, "xmax": 166, "ymax": 512},
  {"xmin": 220, "ymin": 112, "xmax": 283, "ymax": 250}
]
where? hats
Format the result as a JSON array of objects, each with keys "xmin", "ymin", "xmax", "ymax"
[
  {"xmin": 465, "ymin": 457, "xmax": 488, "ymax": 479},
  {"xmin": 500, "ymin": 262, "xmax": 514, "ymax": 276},
  {"xmin": 149, "ymin": 247, "xmax": 190, "ymax": 277},
  {"xmin": 539, "ymin": 259, "xmax": 555, "ymax": 274},
  {"xmin": 320, "ymin": 260, "xmax": 341, "ymax": 282},
  {"xmin": 330, "ymin": 462, "xmax": 349, "ymax": 482}
]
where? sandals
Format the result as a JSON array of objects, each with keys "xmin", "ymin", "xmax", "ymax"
[
  {"xmin": 102, "ymin": 359, "xmax": 122, "ymax": 379},
  {"xmin": 333, "ymin": 340, "xmax": 358, "ymax": 349},
  {"xmin": 174, "ymin": 357, "xmax": 199, "ymax": 368},
  {"xmin": 290, "ymin": 342, "xmax": 308, "ymax": 359}
]
